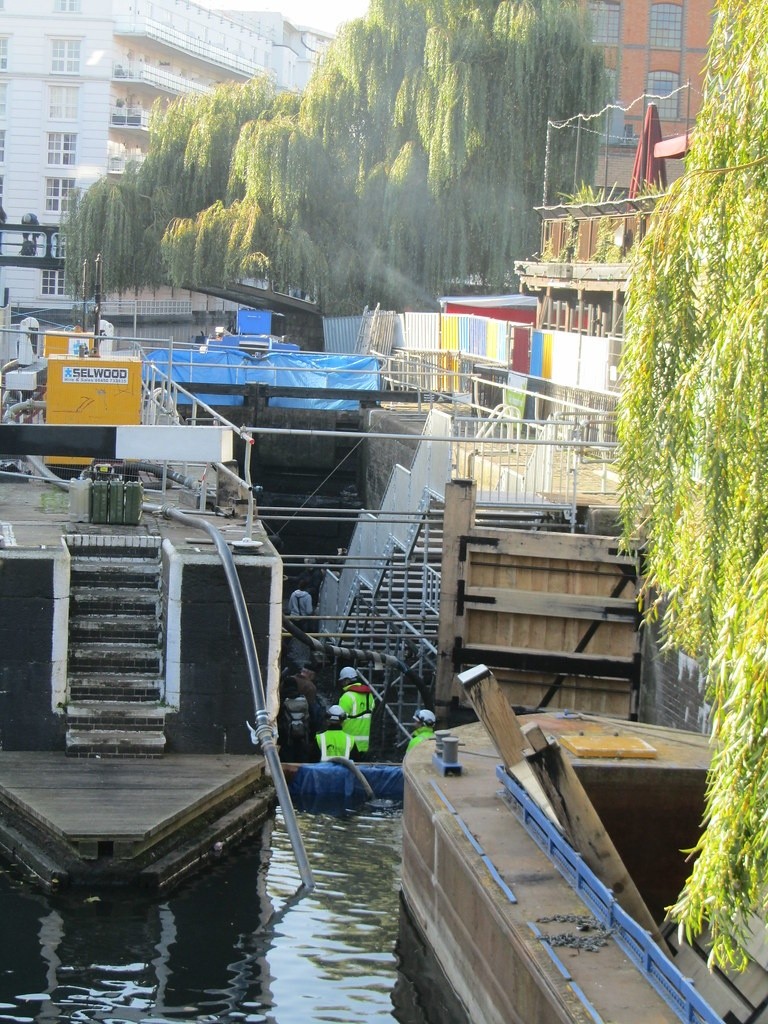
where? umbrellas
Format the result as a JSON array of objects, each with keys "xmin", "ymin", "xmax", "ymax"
[{"xmin": 628, "ymin": 103, "xmax": 667, "ymax": 211}]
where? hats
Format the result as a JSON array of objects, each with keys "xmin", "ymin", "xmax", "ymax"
[
  {"xmin": 299, "ymin": 578, "xmax": 310, "ymax": 589},
  {"xmin": 283, "ymin": 676, "xmax": 296, "ymax": 690},
  {"xmin": 304, "ymin": 663, "xmax": 316, "ymax": 671}
]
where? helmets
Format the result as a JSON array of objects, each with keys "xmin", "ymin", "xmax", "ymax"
[
  {"xmin": 326, "ymin": 705, "xmax": 345, "ymax": 720},
  {"xmin": 412, "ymin": 709, "xmax": 435, "ymax": 728},
  {"xmin": 339, "ymin": 667, "xmax": 357, "ymax": 681}
]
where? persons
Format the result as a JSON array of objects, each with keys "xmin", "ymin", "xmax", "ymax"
[
  {"xmin": 338, "ymin": 667, "xmax": 375, "ymax": 763},
  {"xmin": 406, "ymin": 710, "xmax": 436, "ymax": 755},
  {"xmin": 281, "ymin": 664, "xmax": 318, "ymax": 763},
  {"xmin": 314, "ymin": 706, "xmax": 361, "ymax": 763},
  {"xmin": 288, "ymin": 557, "xmax": 340, "ymax": 632}
]
